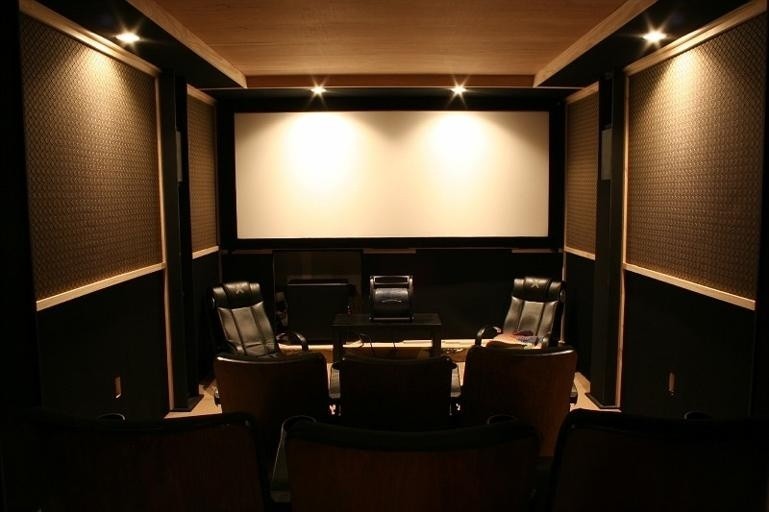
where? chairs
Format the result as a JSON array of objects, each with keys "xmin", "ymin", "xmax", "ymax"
[
  {"xmin": 206, "ymin": 281, "xmax": 309, "ymax": 359},
  {"xmin": 3, "ymin": 407, "xmax": 769, "ymax": 512},
  {"xmin": 186, "ymin": 349, "xmax": 600, "ymax": 457},
  {"xmin": 474, "ymin": 275, "xmax": 570, "ymax": 350},
  {"xmin": 367, "ymin": 273, "xmax": 416, "ymax": 314}
]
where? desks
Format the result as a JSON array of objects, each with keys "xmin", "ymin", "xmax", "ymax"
[{"xmin": 329, "ymin": 312, "xmax": 444, "ymax": 363}]
